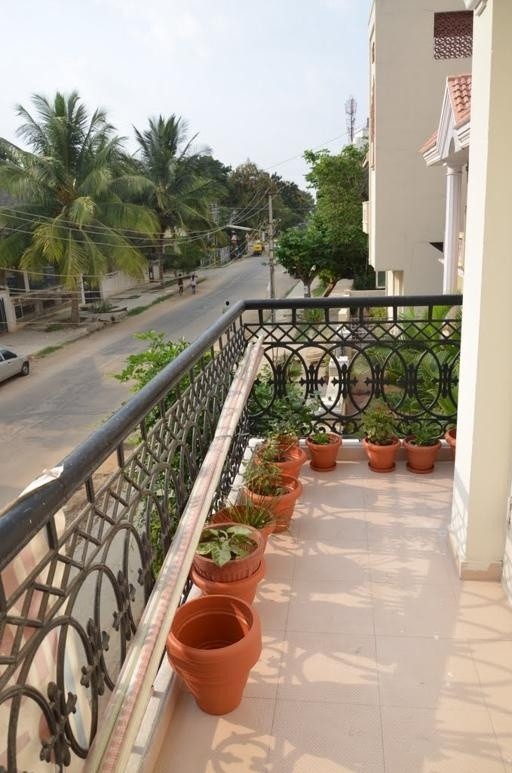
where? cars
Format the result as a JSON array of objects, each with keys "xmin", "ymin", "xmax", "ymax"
[{"xmin": 0, "ymin": 348, "xmax": 29, "ymax": 383}]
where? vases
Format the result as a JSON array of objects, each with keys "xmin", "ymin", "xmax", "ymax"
[
  {"xmin": 445, "ymin": 427, "xmax": 455, "ymax": 455},
  {"xmin": 167, "ymin": 595, "xmax": 262, "ymax": 716}
]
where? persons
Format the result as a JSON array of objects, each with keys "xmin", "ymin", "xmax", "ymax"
[
  {"xmin": 223, "ymin": 301, "xmax": 232, "ymax": 334},
  {"xmin": 177, "ymin": 274, "xmax": 183, "ymax": 295},
  {"xmin": 191, "ymin": 275, "xmax": 196, "ymax": 294}
]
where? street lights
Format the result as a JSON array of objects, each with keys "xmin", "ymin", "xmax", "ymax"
[{"xmin": 226, "ymin": 225, "xmax": 275, "ymax": 327}]
